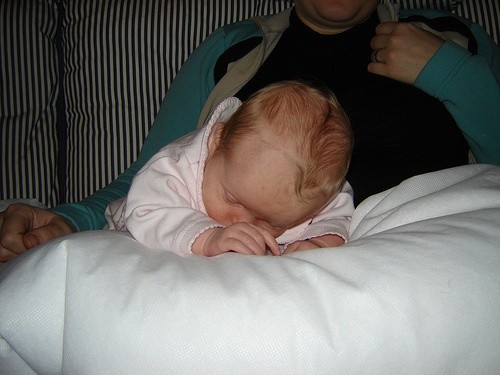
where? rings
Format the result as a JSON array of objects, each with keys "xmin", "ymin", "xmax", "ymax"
[{"xmin": 374, "ymin": 48, "xmax": 381, "ymax": 63}]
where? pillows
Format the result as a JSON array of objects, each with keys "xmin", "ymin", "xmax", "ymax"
[{"xmin": 0, "ymin": 161, "xmax": 500, "ymax": 375}]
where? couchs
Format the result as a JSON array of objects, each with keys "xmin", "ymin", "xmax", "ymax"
[{"xmin": 0, "ymin": 0, "xmax": 500, "ymax": 210}]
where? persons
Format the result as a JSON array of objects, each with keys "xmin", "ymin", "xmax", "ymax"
[
  {"xmin": 0, "ymin": 0, "xmax": 499, "ymax": 266},
  {"xmin": 103, "ymin": 79, "xmax": 354, "ymax": 260}
]
